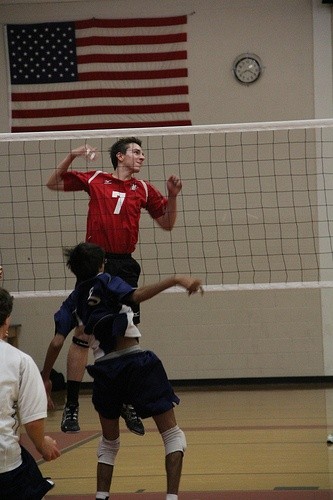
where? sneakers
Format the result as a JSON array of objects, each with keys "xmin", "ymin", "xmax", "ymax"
[
  {"xmin": 120, "ymin": 404, "xmax": 145, "ymax": 436},
  {"xmin": 61, "ymin": 403, "xmax": 80, "ymax": 432}
]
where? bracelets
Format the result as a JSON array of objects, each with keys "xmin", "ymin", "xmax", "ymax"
[{"xmin": 42, "ymin": 363, "xmax": 53, "ymax": 376}]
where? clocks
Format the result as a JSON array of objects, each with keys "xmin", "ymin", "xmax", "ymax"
[{"xmin": 233, "ymin": 52, "xmax": 265, "ymax": 87}]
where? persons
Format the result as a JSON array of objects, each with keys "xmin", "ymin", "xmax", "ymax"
[
  {"xmin": 0, "ymin": 286, "xmax": 60, "ymax": 500},
  {"xmin": 41, "ymin": 242, "xmax": 204, "ymax": 500},
  {"xmin": 45, "ymin": 135, "xmax": 182, "ymax": 436}
]
